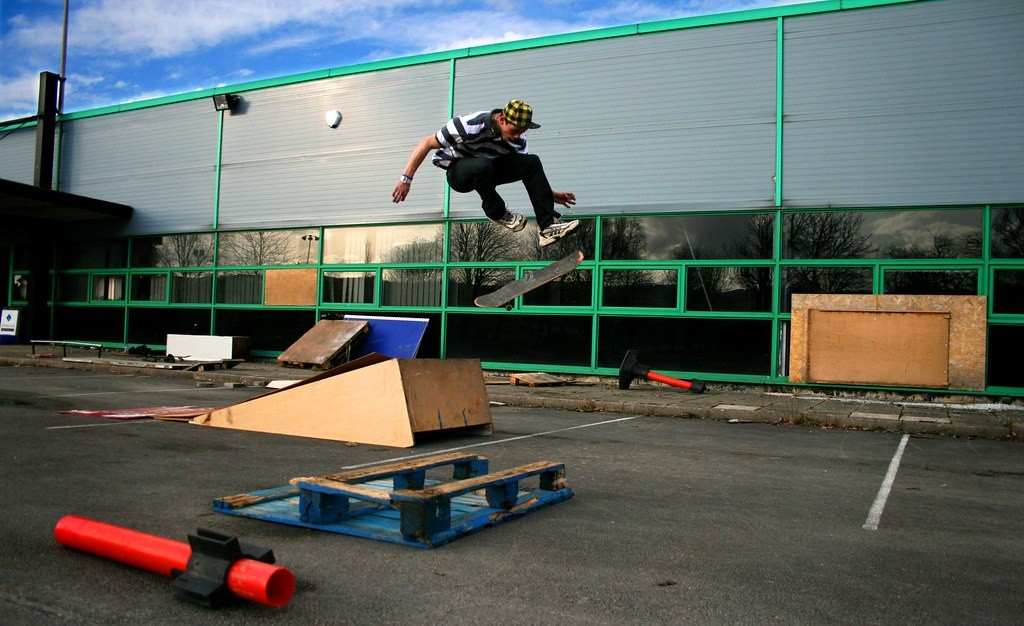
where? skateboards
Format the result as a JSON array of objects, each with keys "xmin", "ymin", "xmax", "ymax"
[{"xmin": 473, "ymin": 251, "xmax": 583, "ymax": 311}]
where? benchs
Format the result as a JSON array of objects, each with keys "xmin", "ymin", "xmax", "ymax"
[{"xmin": 30, "ymin": 340, "xmax": 103, "ymax": 358}]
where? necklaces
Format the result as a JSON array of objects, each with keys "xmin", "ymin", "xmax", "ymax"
[{"xmin": 494, "ymin": 114, "xmax": 500, "ymax": 123}]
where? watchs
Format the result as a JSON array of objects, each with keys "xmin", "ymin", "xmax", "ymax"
[{"xmin": 400, "ymin": 174, "xmax": 413, "ymax": 184}]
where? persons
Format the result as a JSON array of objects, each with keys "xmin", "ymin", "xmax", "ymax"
[{"xmin": 392, "ymin": 99, "xmax": 582, "ymax": 248}]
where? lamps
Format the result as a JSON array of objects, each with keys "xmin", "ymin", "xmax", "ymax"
[{"xmin": 211, "ymin": 93, "xmax": 240, "ymax": 111}]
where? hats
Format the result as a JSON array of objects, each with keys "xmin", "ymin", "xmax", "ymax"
[{"xmin": 502, "ymin": 100, "xmax": 541, "ymax": 129}]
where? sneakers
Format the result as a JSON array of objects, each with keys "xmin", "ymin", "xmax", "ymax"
[
  {"xmin": 488, "ymin": 208, "xmax": 528, "ymax": 232},
  {"xmin": 538, "ymin": 219, "xmax": 582, "ymax": 247}
]
